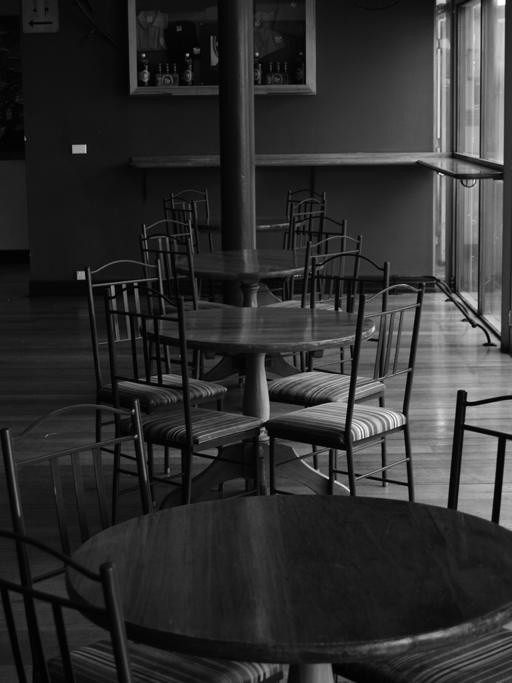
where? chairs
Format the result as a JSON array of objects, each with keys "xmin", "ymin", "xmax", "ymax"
[
  {"xmin": 267, "ymin": 252, "xmax": 391, "ymax": 486},
  {"xmin": 0, "ymin": 529, "xmax": 133, "ymax": 683},
  {"xmin": 261, "ymin": 233, "xmax": 363, "ymax": 372},
  {"xmin": 283, "ymin": 188, "xmax": 325, "ymax": 249},
  {"xmin": 265, "ymin": 282, "xmax": 426, "ymax": 503},
  {"xmin": 162, "ymin": 197, "xmax": 214, "ymax": 301},
  {"xmin": 84, "ymin": 259, "xmax": 228, "ymax": 500},
  {"xmin": 139, "ymin": 233, "xmax": 239, "ymax": 382},
  {"xmin": 288, "ymin": 198, "xmax": 326, "ymax": 251},
  {"xmin": 332, "ymin": 627, "xmax": 512, "ymax": 682},
  {"xmin": 140, "ymin": 217, "xmax": 211, "ymax": 367},
  {"xmin": 103, "ymin": 286, "xmax": 265, "ymax": 523},
  {"xmin": 448, "ymin": 390, "xmax": 512, "ymax": 525},
  {"xmin": 290, "ymin": 215, "xmax": 348, "ymax": 367},
  {"xmin": 170, "ymin": 185, "xmax": 214, "ymax": 253},
  {"xmin": 0, "ymin": 398, "xmax": 284, "ymax": 683}
]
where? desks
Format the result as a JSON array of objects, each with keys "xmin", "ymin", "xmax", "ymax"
[
  {"xmin": 185, "ymin": 217, "xmax": 303, "ymax": 306},
  {"xmin": 139, "ymin": 308, "xmax": 376, "ymax": 508},
  {"xmin": 176, "ymin": 248, "xmax": 325, "ymax": 382},
  {"xmin": 65, "ymin": 494, "xmax": 512, "ymax": 683}
]
